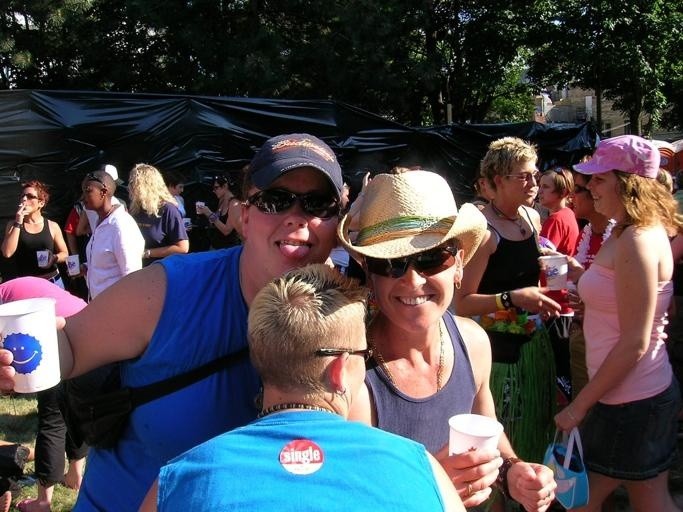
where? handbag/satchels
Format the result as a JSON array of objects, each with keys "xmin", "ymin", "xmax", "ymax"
[
  {"xmin": 542, "ymin": 444, "xmax": 589, "ymax": 510},
  {"xmin": 54, "ymin": 361, "xmax": 131, "ymax": 448},
  {"xmin": 486, "ymin": 331, "xmax": 521, "ymax": 365}
]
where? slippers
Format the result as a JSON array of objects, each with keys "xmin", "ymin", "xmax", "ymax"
[{"xmin": 15, "ymin": 499, "xmax": 52, "ymax": 512}]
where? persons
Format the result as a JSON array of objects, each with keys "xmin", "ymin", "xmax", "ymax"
[
  {"xmin": 455, "ymin": 134, "xmax": 682, "ymax": 512},
  {"xmin": 0, "ymin": 133, "xmax": 371, "ymax": 511},
  {"xmin": 137, "ymin": 264, "xmax": 468, "ymax": 512},
  {"xmin": 334, "ymin": 169, "xmax": 557, "ymax": 512}
]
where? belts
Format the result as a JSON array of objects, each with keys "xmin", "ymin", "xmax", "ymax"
[{"xmin": 50, "ymin": 273, "xmax": 60, "ymax": 284}]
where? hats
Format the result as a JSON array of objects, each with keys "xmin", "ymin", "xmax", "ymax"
[
  {"xmin": 338, "ymin": 171, "xmax": 487, "ymax": 269},
  {"xmin": 573, "ymin": 134, "xmax": 661, "ymax": 178},
  {"xmin": 102, "ymin": 164, "xmax": 124, "ymax": 186},
  {"xmin": 245, "ymin": 134, "xmax": 343, "ymax": 201}
]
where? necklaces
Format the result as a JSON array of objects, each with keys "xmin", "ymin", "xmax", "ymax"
[{"xmin": 370, "ymin": 320, "xmax": 445, "ymax": 392}]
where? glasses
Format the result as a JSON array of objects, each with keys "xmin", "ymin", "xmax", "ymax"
[
  {"xmin": 213, "ymin": 186, "xmax": 220, "ymax": 190},
  {"xmin": 20, "ymin": 193, "xmax": 38, "ymax": 199},
  {"xmin": 574, "ymin": 183, "xmax": 587, "ymax": 196},
  {"xmin": 507, "ymin": 170, "xmax": 541, "ymax": 182},
  {"xmin": 552, "ymin": 165, "xmax": 564, "ymax": 180},
  {"xmin": 87, "ymin": 171, "xmax": 107, "ymax": 187},
  {"xmin": 246, "ymin": 188, "xmax": 341, "ymax": 219},
  {"xmin": 316, "ymin": 343, "xmax": 373, "ymax": 366},
  {"xmin": 367, "ymin": 246, "xmax": 457, "ymax": 279}
]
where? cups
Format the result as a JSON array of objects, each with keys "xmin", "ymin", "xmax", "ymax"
[
  {"xmin": 183, "ymin": 218, "xmax": 190, "ymax": 230},
  {"xmin": 448, "ymin": 414, "xmax": 505, "ymax": 458},
  {"xmin": 36, "ymin": 250, "xmax": 49, "ymax": 267},
  {"xmin": 0, "ymin": 297, "xmax": 61, "ymax": 394},
  {"xmin": 196, "ymin": 202, "xmax": 205, "ymax": 214},
  {"xmin": 539, "ymin": 255, "xmax": 569, "ymax": 291},
  {"xmin": 64, "ymin": 254, "xmax": 81, "ymax": 275}
]
